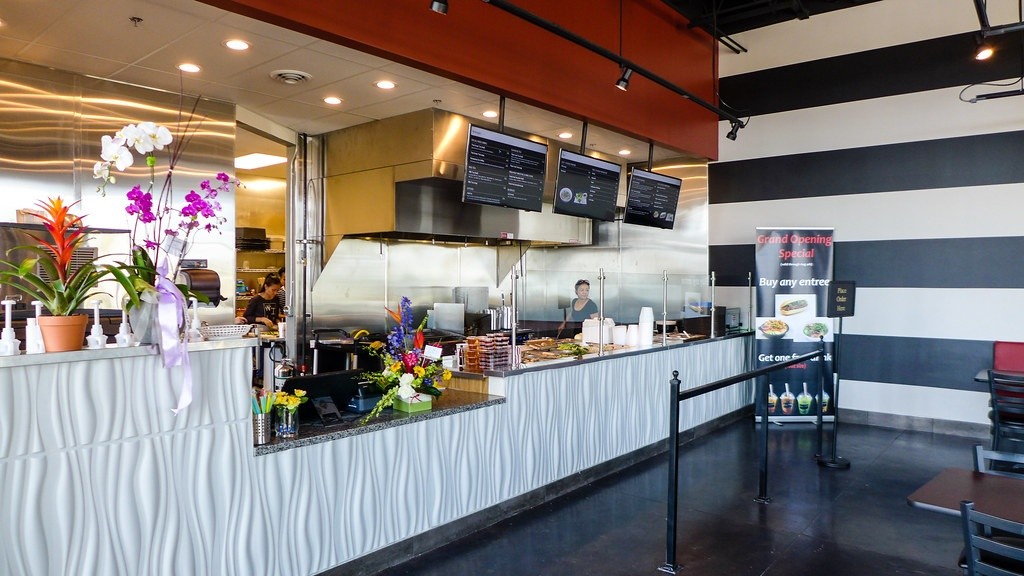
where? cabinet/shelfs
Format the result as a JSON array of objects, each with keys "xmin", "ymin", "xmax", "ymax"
[{"xmin": 237, "ymin": 249, "xmax": 285, "ymax": 300}]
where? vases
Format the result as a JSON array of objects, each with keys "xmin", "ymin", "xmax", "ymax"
[
  {"xmin": 129, "ymin": 300, "xmax": 177, "ymax": 344},
  {"xmin": 275, "ymin": 408, "xmax": 299, "ymax": 438}
]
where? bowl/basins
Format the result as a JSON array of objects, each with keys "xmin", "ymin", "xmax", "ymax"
[
  {"xmin": 612, "ymin": 307, "xmax": 654, "ymax": 346},
  {"xmin": 758, "ymin": 322, "xmax": 789, "ymax": 339}
]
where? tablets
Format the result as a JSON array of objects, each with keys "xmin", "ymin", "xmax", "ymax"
[{"xmin": 269, "ymin": 368, "xmax": 364, "ymax": 427}]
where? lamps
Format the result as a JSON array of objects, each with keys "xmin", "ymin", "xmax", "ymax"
[
  {"xmin": 615, "ymin": 68, "xmax": 632, "ymax": 91},
  {"xmin": 726, "ymin": 124, "xmax": 740, "ymax": 140},
  {"xmin": 976, "ymin": 31, "xmax": 992, "ymax": 62},
  {"xmin": 429, "ymin": 0, "xmax": 448, "ymax": 13}
]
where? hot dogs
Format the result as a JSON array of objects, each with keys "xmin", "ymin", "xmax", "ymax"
[{"xmin": 780, "ymin": 299, "xmax": 808, "ymax": 315}]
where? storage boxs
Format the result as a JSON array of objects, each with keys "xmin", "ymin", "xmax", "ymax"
[
  {"xmin": 236, "ymin": 308, "xmax": 246, "ymax": 316},
  {"xmin": 393, "ymin": 392, "xmax": 432, "ymax": 413}
]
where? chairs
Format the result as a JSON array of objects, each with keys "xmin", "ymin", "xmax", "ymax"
[{"xmin": 959, "ymin": 341, "xmax": 1024, "ymax": 576}]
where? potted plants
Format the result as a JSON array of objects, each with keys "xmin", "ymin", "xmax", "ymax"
[{"xmin": 0, "ymin": 196, "xmax": 161, "ymax": 353}]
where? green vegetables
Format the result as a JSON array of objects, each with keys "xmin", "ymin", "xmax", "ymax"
[
  {"xmin": 558, "ymin": 343, "xmax": 590, "ymax": 355},
  {"xmin": 804, "ymin": 323, "xmax": 827, "ymax": 335}
]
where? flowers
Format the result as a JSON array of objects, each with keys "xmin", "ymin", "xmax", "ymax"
[
  {"xmin": 270, "ymin": 388, "xmax": 309, "ymax": 415},
  {"xmin": 351, "ymin": 297, "xmax": 452, "ymax": 425},
  {"xmin": 92, "ymin": 69, "xmax": 246, "ymax": 416}
]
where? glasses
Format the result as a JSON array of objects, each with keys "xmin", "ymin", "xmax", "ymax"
[{"xmin": 576, "ymin": 280, "xmax": 589, "ymax": 285}]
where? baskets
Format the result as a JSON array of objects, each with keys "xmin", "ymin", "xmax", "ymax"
[{"xmin": 197, "ymin": 325, "xmax": 253, "ymax": 339}]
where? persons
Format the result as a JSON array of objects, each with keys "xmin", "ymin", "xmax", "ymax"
[
  {"xmin": 557, "ymin": 279, "xmax": 598, "ymax": 337},
  {"xmin": 242, "ymin": 267, "xmax": 286, "ymax": 329}
]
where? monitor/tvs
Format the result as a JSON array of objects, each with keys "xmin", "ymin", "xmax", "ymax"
[
  {"xmin": 461, "ymin": 124, "xmax": 549, "ymax": 212},
  {"xmin": 552, "ymin": 148, "xmax": 622, "ymax": 223},
  {"xmin": 621, "ymin": 166, "xmax": 681, "ymax": 230}
]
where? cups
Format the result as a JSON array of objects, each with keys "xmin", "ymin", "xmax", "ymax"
[
  {"xmin": 768, "ymin": 393, "xmax": 778, "ymax": 413},
  {"xmin": 797, "ymin": 392, "xmax": 812, "ymax": 415},
  {"xmin": 278, "ymin": 322, "xmax": 285, "ymax": 338},
  {"xmin": 815, "ymin": 392, "xmax": 830, "ymax": 412},
  {"xmin": 780, "ymin": 392, "xmax": 795, "ymax": 415}
]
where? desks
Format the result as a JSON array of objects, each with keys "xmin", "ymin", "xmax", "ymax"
[
  {"xmin": 974, "ymin": 368, "xmax": 1024, "ymax": 387},
  {"xmin": 906, "ymin": 467, "xmax": 1024, "ymax": 537}
]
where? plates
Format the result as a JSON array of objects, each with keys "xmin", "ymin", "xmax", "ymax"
[{"xmin": 196, "ymin": 325, "xmax": 254, "ymax": 341}]
